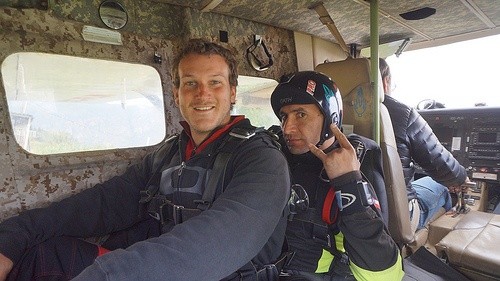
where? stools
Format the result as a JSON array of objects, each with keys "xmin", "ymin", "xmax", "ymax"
[{"xmin": 434, "ymin": 209, "xmax": 500, "ymax": 281}]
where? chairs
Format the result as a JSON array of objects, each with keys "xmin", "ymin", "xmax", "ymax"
[{"xmin": 315, "ymin": 58, "xmax": 448, "ymax": 256}]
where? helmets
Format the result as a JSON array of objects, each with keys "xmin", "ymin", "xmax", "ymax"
[{"xmin": 270, "ymin": 71, "xmax": 344, "ymax": 140}]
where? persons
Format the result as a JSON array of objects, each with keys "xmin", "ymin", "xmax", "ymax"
[
  {"xmin": 365, "ymin": 54, "xmax": 474, "ymax": 239},
  {"xmin": 261, "ymin": 68, "xmax": 408, "ymax": 281},
  {"xmin": 0, "ymin": 35, "xmax": 291, "ymax": 280}
]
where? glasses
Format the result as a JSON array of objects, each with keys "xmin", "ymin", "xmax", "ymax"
[{"xmin": 247, "ymin": 39, "xmax": 273, "ymax": 72}]
